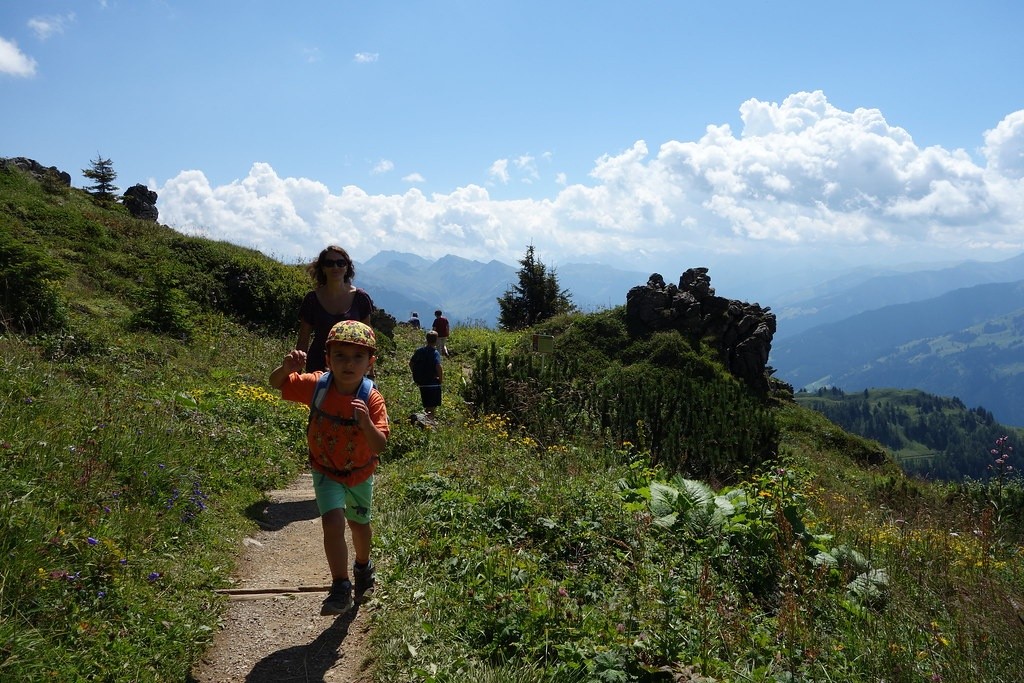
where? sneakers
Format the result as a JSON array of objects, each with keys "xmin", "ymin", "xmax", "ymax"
[
  {"xmin": 353, "ymin": 560, "xmax": 376, "ymax": 605},
  {"xmin": 320, "ymin": 581, "xmax": 352, "ymax": 615}
]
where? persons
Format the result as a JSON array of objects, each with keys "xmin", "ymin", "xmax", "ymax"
[
  {"xmin": 295, "ymin": 245, "xmax": 377, "ymax": 392},
  {"xmin": 431, "ymin": 311, "xmax": 450, "ymax": 360},
  {"xmin": 269, "ymin": 322, "xmax": 389, "ymax": 614},
  {"xmin": 409, "ymin": 330, "xmax": 443, "ymax": 425},
  {"xmin": 407, "ymin": 312, "xmax": 423, "ymax": 330}
]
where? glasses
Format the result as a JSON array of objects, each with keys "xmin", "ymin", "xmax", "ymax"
[{"xmin": 323, "ymin": 259, "xmax": 347, "ymax": 267}]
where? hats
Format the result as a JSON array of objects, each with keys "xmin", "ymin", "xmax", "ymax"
[{"xmin": 325, "ymin": 320, "xmax": 377, "ymax": 352}]
